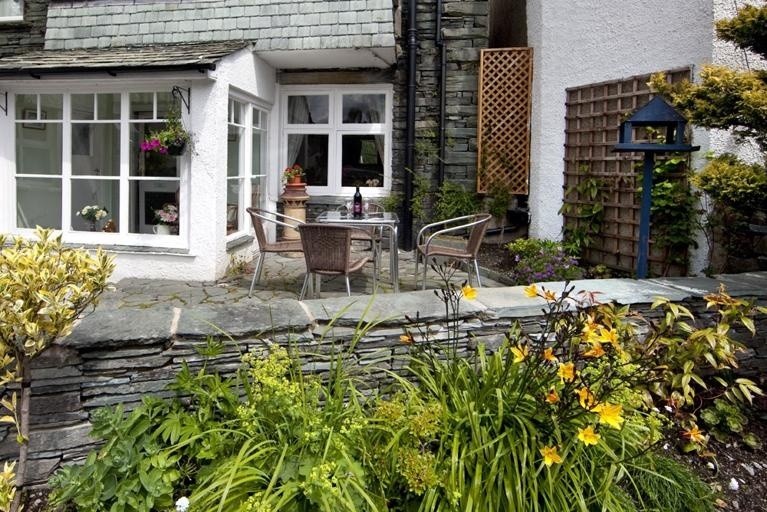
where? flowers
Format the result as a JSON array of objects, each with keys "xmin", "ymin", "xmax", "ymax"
[
  {"xmin": 150, "ymin": 202, "xmax": 178, "ymax": 225},
  {"xmin": 283, "ymin": 165, "xmax": 306, "ymax": 180},
  {"xmin": 76, "ymin": 205, "xmax": 109, "ymax": 231},
  {"xmin": 140, "ymin": 105, "xmax": 196, "ymax": 157}
]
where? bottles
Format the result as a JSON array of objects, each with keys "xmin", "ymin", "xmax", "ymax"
[{"xmin": 353, "ymin": 186, "xmax": 363, "ymax": 220}]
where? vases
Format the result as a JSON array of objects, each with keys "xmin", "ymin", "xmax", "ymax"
[
  {"xmin": 288, "ymin": 175, "xmax": 301, "ymax": 183},
  {"xmin": 168, "ymin": 144, "xmax": 186, "ymax": 155}
]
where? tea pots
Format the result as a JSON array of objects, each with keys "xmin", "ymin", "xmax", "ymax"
[{"xmin": 151, "ymin": 223, "xmax": 175, "ymax": 236}]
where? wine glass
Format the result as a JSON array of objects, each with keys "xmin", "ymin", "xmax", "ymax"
[
  {"xmin": 345, "ymin": 198, "xmax": 353, "ymax": 217},
  {"xmin": 363, "ymin": 199, "xmax": 370, "ymax": 217}
]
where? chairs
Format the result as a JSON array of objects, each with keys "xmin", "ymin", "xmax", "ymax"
[{"xmin": 245, "ymin": 202, "xmax": 492, "ymax": 302}]
